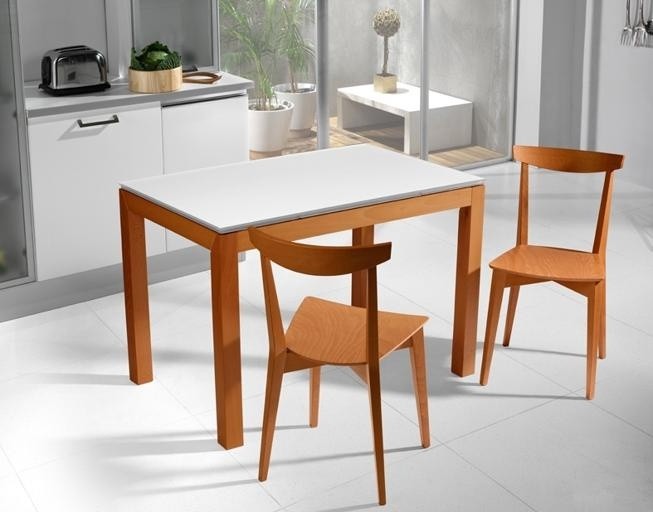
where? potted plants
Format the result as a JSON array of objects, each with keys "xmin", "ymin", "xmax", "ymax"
[
  {"xmin": 213, "ymin": 2, "xmax": 296, "ymax": 157},
  {"xmin": 373, "ymin": 7, "xmax": 401, "ymax": 95},
  {"xmin": 270, "ymin": 0, "xmax": 319, "ymax": 134}
]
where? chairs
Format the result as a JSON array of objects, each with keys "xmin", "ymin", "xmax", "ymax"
[
  {"xmin": 475, "ymin": 140, "xmax": 630, "ymax": 402},
  {"xmin": 241, "ymin": 220, "xmax": 440, "ymax": 507}
]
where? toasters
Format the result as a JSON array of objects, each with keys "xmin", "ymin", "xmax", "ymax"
[{"xmin": 37, "ymin": 44, "xmax": 111, "ymax": 96}]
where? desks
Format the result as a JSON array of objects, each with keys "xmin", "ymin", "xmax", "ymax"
[
  {"xmin": 334, "ymin": 75, "xmax": 480, "ymax": 159},
  {"xmin": 113, "ymin": 137, "xmax": 489, "ymax": 455}
]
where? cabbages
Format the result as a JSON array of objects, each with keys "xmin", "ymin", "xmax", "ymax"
[{"xmin": 130, "ymin": 40, "xmax": 181, "ymax": 71}]
